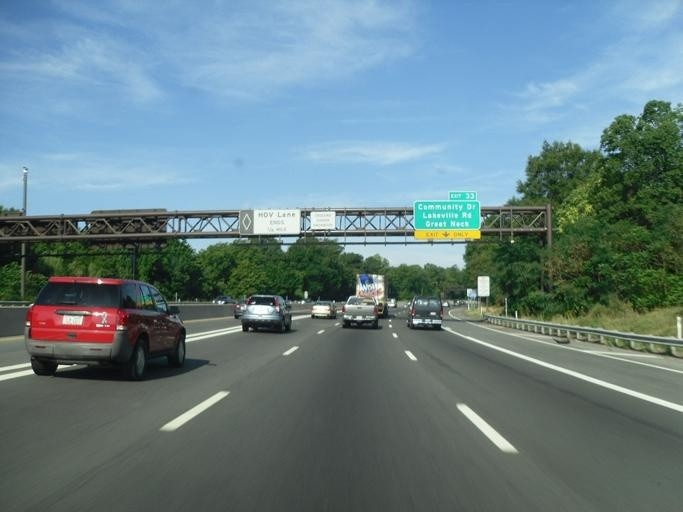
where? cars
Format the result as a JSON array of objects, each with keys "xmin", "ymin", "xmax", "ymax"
[
  {"xmin": 232, "ymin": 300, "xmax": 247, "ymax": 318},
  {"xmin": 310, "ymin": 300, "xmax": 336, "ymax": 319},
  {"xmin": 212, "ymin": 296, "xmax": 235, "ymax": 305},
  {"xmin": 385, "ymin": 295, "xmax": 470, "ymax": 331}
]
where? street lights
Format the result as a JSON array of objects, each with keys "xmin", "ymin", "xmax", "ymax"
[{"xmin": 19, "ymin": 167, "xmax": 30, "ymax": 299}]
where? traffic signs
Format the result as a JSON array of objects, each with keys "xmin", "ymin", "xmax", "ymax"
[
  {"xmin": 238, "ymin": 209, "xmax": 300, "ymax": 235},
  {"xmin": 449, "ymin": 192, "xmax": 477, "ymax": 201},
  {"xmin": 412, "ymin": 200, "xmax": 481, "ymax": 230},
  {"xmin": 414, "ymin": 230, "xmax": 481, "ymax": 240}
]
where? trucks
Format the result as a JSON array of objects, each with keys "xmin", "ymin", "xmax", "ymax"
[{"xmin": 354, "ymin": 273, "xmax": 388, "ymax": 318}]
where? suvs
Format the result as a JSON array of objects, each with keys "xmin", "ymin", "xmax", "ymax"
[
  {"xmin": 22, "ymin": 276, "xmax": 186, "ymax": 379},
  {"xmin": 342, "ymin": 295, "xmax": 379, "ymax": 328},
  {"xmin": 240, "ymin": 294, "xmax": 292, "ymax": 333}
]
